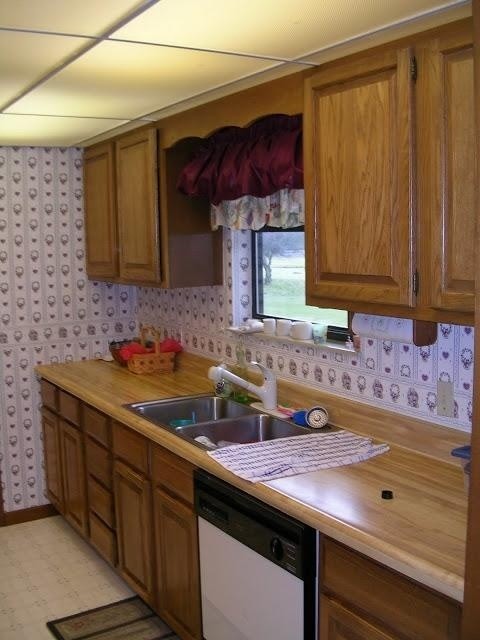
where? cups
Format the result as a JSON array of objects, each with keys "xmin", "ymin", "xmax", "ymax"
[
  {"xmin": 291, "ymin": 322, "xmax": 312, "ymax": 340},
  {"xmin": 312, "ymin": 324, "xmax": 328, "ymax": 344},
  {"xmin": 263, "ymin": 318, "xmax": 275, "ymax": 333},
  {"xmin": 277, "ymin": 319, "xmax": 291, "ymax": 336}
]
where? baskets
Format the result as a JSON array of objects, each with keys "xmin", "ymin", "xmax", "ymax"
[{"xmin": 126, "ymin": 325, "xmax": 175, "ymax": 375}]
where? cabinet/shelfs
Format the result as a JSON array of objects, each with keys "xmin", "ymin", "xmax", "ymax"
[
  {"xmin": 111, "ymin": 408, "xmax": 204, "ymax": 640},
  {"xmin": 304, "ymin": 19, "xmax": 476, "ymax": 324},
  {"xmin": 319, "ymin": 512, "xmax": 464, "ymax": 639},
  {"xmin": 36, "ymin": 370, "xmax": 89, "ymax": 546},
  {"xmin": 84, "ymin": 398, "xmax": 117, "ymax": 572},
  {"xmin": 83, "ymin": 86, "xmax": 224, "ymax": 289}
]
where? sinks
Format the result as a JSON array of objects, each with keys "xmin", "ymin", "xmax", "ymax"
[
  {"xmin": 178, "ymin": 415, "xmax": 313, "ymax": 450},
  {"xmin": 135, "ymin": 395, "xmax": 254, "ymax": 427}
]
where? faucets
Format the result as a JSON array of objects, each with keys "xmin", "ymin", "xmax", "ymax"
[{"xmin": 208, "ymin": 362, "xmax": 278, "ymax": 409}]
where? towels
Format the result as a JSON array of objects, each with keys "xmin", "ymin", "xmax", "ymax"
[{"xmin": 206, "ymin": 429, "xmax": 390, "ymax": 485}]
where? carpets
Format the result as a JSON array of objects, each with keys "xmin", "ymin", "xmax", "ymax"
[{"xmin": 47, "ymin": 595, "xmax": 180, "ymax": 639}]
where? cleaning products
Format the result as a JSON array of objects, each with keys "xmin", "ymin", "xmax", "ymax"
[{"xmin": 212, "ymin": 357, "xmax": 232, "ymax": 397}]
